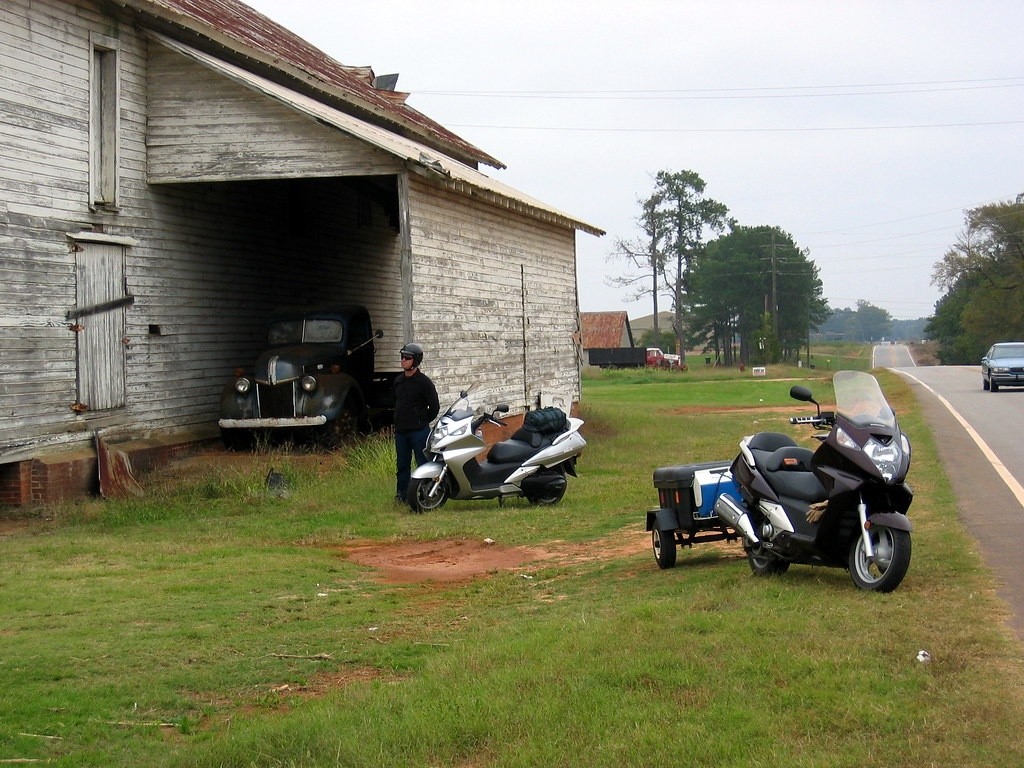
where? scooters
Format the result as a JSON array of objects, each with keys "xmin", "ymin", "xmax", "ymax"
[
  {"xmin": 406, "ymin": 379, "xmax": 587, "ymax": 515},
  {"xmin": 648, "ymin": 369, "xmax": 915, "ymax": 594}
]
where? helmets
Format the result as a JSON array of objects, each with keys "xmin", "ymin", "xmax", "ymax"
[{"xmin": 399, "ymin": 343, "xmax": 423, "ymax": 368}]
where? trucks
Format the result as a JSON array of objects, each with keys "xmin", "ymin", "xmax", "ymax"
[
  {"xmin": 579, "ymin": 347, "xmax": 681, "ymax": 372},
  {"xmin": 218, "ymin": 306, "xmax": 400, "ymax": 452}
]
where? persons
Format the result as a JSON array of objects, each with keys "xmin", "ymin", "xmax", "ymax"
[{"xmin": 391, "ymin": 343, "xmax": 439, "ymax": 506}]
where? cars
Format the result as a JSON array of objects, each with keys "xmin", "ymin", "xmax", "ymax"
[{"xmin": 981, "ymin": 342, "xmax": 1024, "ymax": 392}]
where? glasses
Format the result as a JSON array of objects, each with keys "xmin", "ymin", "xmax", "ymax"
[{"xmin": 401, "ymin": 354, "xmax": 413, "ymax": 360}]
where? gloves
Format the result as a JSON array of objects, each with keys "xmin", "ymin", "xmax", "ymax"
[{"xmin": 805, "ymin": 499, "xmax": 829, "ymax": 524}]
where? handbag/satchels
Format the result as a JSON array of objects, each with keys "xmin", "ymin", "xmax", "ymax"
[{"xmin": 522, "ymin": 406, "xmax": 566, "ymax": 434}]
